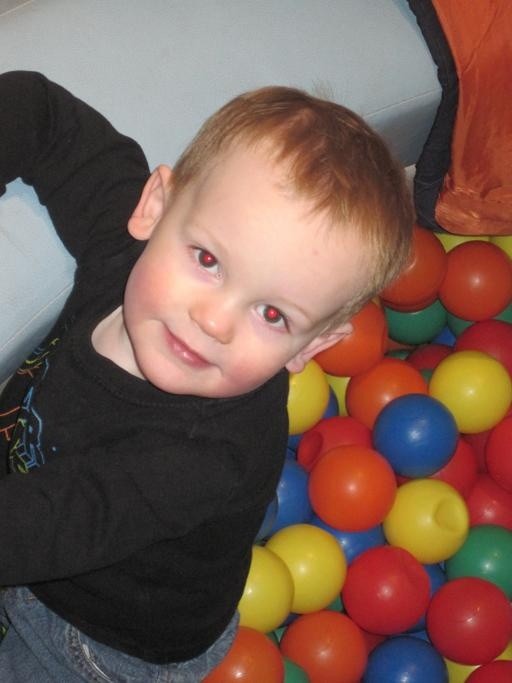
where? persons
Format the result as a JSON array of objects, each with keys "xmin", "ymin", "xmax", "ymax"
[{"xmin": 0, "ymin": 68, "xmax": 414, "ymax": 683}]
